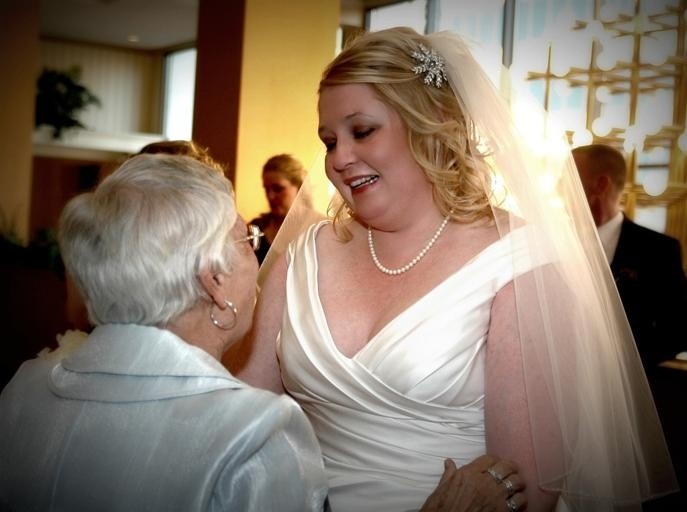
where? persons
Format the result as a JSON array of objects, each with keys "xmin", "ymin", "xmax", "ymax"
[
  {"xmin": 1, "ymin": 152, "xmax": 528, "ymax": 512},
  {"xmin": 65, "ymin": 140, "xmax": 214, "ymax": 332},
  {"xmin": 569, "ymin": 144, "xmax": 687, "ymax": 386},
  {"xmin": 246, "ymin": 155, "xmax": 303, "ymax": 267},
  {"xmin": 220, "ymin": 25, "xmax": 675, "ymax": 512}
]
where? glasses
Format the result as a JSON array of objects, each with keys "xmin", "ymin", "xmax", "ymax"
[{"xmin": 235, "ymin": 224, "xmax": 265, "ymax": 252}]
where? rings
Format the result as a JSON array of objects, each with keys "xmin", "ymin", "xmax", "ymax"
[
  {"xmin": 503, "ymin": 479, "xmax": 515, "ymax": 496},
  {"xmin": 507, "ymin": 498, "xmax": 520, "ymax": 512},
  {"xmin": 486, "ymin": 469, "xmax": 503, "ymax": 484}
]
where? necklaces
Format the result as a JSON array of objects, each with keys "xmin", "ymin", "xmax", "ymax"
[{"xmin": 366, "ymin": 203, "xmax": 454, "ymax": 276}]
